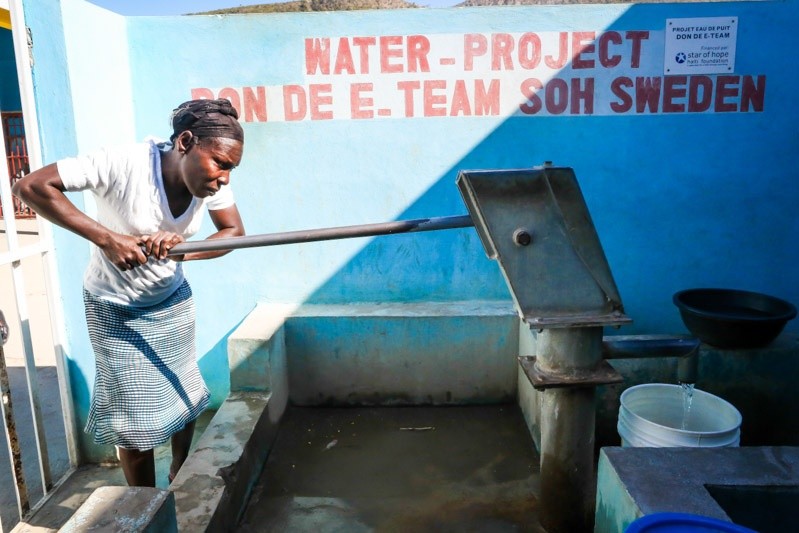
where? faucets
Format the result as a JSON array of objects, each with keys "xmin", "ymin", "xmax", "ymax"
[{"xmin": 601, "ymin": 339, "xmax": 698, "ymax": 386}]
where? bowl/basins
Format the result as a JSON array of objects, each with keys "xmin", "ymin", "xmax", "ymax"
[{"xmin": 671, "ymin": 285, "xmax": 799, "ymax": 350}]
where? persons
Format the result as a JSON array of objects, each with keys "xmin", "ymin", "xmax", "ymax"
[{"xmin": 12, "ymin": 95, "xmax": 246, "ymax": 487}]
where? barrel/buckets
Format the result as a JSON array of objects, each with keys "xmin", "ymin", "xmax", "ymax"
[{"xmin": 615, "ymin": 382, "xmax": 741, "ymax": 459}]
